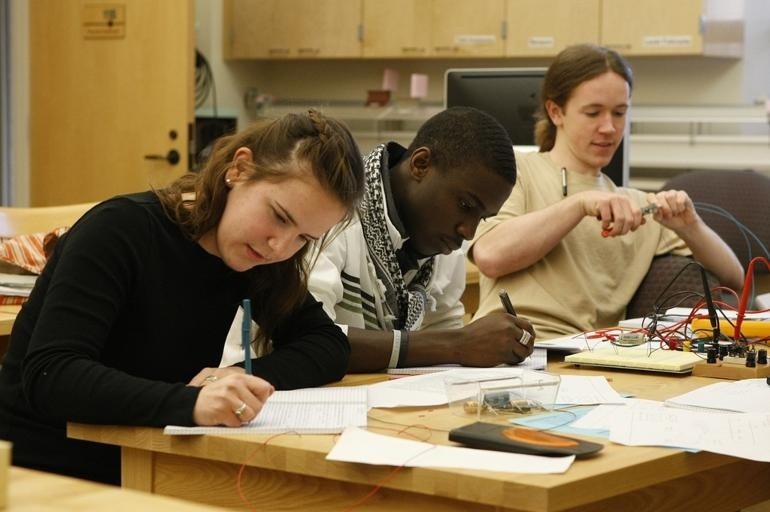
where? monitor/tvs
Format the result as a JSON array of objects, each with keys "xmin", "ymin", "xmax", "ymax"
[{"xmin": 444, "ymin": 67, "xmax": 629, "ymax": 193}]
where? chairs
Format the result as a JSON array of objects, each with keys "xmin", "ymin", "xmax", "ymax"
[{"xmin": 624, "ymin": 167, "xmax": 769, "ymax": 318}]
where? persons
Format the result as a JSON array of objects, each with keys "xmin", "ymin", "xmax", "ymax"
[
  {"xmin": 458, "ymin": 43, "xmax": 748, "ymax": 361},
  {"xmin": 0, "ymin": 106, "xmax": 368, "ymax": 490},
  {"xmin": 215, "ymin": 101, "xmax": 539, "ymax": 374}
]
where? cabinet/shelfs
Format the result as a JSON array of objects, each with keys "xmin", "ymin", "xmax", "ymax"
[{"xmin": 222, "ymin": 0, "xmax": 707, "ymax": 63}]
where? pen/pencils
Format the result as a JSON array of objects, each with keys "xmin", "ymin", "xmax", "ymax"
[
  {"xmin": 243, "ymin": 298, "xmax": 252, "ymax": 375},
  {"xmin": 498, "ymin": 289, "xmax": 519, "ymax": 316}
]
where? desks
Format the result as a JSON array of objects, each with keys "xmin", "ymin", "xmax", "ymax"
[{"xmin": 66, "ymin": 336, "xmax": 769, "ymax": 511}]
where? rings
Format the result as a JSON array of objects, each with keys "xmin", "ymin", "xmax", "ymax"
[
  {"xmin": 204, "ymin": 374, "xmax": 219, "ymax": 383},
  {"xmin": 232, "ymin": 402, "xmax": 247, "ymax": 417},
  {"xmin": 518, "ymin": 329, "xmax": 532, "ymax": 346}
]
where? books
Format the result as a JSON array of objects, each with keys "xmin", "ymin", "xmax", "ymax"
[
  {"xmin": 564, "ymin": 344, "xmax": 711, "ymax": 377},
  {"xmin": 617, "ymin": 306, "xmax": 770, "ymax": 339},
  {"xmin": 385, "ymin": 347, "xmax": 551, "ymax": 375},
  {"xmin": 441, "ymin": 377, "xmax": 560, "ymax": 397},
  {"xmin": 0, "ymin": 285, "xmax": 34, "ymax": 297},
  {"xmin": 1, "ymin": 271, "xmax": 39, "ymax": 289},
  {"xmin": 162, "ymin": 382, "xmax": 381, "ymax": 437},
  {"xmin": 0, "ymin": 295, "xmax": 30, "ymax": 306},
  {"xmin": 0, "ymin": 305, "xmax": 23, "ymax": 315}
]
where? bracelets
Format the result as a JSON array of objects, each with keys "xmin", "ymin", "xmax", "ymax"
[{"xmin": 388, "ymin": 327, "xmax": 410, "ymax": 369}]
